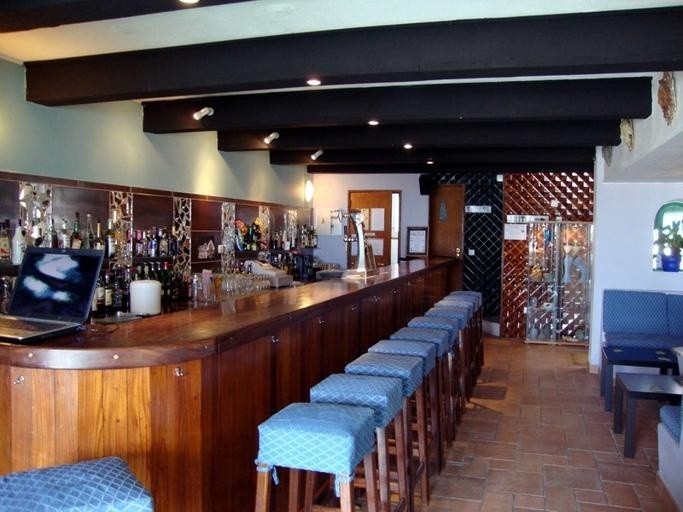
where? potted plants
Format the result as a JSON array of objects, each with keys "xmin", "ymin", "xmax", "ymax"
[{"xmin": 654, "ymin": 220, "xmax": 683, "ymax": 272}]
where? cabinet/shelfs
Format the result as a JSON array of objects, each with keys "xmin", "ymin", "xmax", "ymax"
[{"xmin": 524, "ymin": 221, "xmax": 592, "ymax": 348}]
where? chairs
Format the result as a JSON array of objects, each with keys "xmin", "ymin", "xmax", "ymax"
[{"xmin": 598, "ymin": 288, "xmax": 683, "ymax": 510}]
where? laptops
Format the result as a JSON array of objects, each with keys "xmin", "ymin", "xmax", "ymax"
[{"xmin": 0, "ymin": 246, "xmax": 106, "ymax": 343}]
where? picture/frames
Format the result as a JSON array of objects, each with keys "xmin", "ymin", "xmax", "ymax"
[{"xmin": 406, "ymin": 226, "xmax": 427, "ymax": 255}]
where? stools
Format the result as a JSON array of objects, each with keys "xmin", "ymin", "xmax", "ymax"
[
  {"xmin": 0, "ymin": 454, "xmax": 154, "ymax": 512},
  {"xmin": 450, "ymin": 291, "xmax": 483, "ymax": 360},
  {"xmin": 252, "ymin": 401, "xmax": 382, "ymax": 511},
  {"xmin": 434, "ymin": 299, "xmax": 475, "ymax": 397},
  {"xmin": 343, "ymin": 351, "xmax": 432, "ymax": 507},
  {"xmin": 407, "ymin": 313, "xmax": 461, "ymax": 431},
  {"xmin": 442, "ymin": 295, "xmax": 480, "ymax": 376},
  {"xmin": 424, "ymin": 305, "xmax": 470, "ymax": 416},
  {"xmin": 367, "ymin": 338, "xmax": 442, "ymax": 483},
  {"xmin": 388, "ymin": 326, "xmax": 453, "ymax": 454},
  {"xmin": 308, "ymin": 372, "xmax": 410, "ymax": 511}
]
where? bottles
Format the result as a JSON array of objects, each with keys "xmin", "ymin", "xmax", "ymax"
[
  {"xmin": 235, "ymin": 223, "xmax": 318, "ymax": 281},
  {"xmin": 0, "ymin": 210, "xmax": 173, "ymax": 320}
]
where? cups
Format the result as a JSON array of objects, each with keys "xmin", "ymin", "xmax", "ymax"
[{"xmin": 189, "ymin": 269, "xmax": 273, "ymax": 302}]
